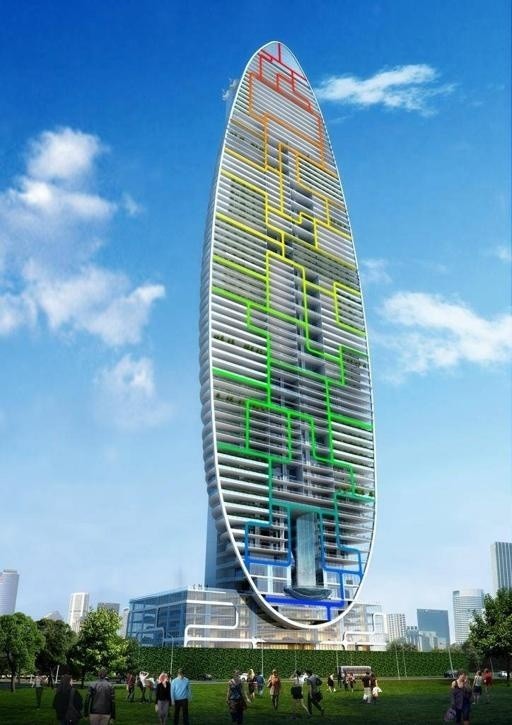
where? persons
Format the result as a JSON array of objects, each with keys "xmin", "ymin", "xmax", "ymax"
[
  {"xmin": 481, "ymin": 668, "xmax": 492, "ymax": 705},
  {"xmin": 349, "ymin": 672, "xmax": 356, "ymax": 692},
  {"xmin": 335, "ymin": 671, "xmax": 342, "ymax": 688},
  {"xmin": 115, "ymin": 668, "xmax": 192, "ymax": 725},
  {"xmin": 226, "ymin": 666, "xmax": 326, "ymax": 725},
  {"xmin": 361, "ymin": 671, "xmax": 372, "ymax": 705},
  {"xmin": 327, "ymin": 672, "xmax": 336, "ymax": 692},
  {"xmin": 470, "ymin": 671, "xmax": 484, "ymax": 705},
  {"xmin": 53, "ymin": 674, "xmax": 84, "ymax": 725},
  {"xmin": 343, "ymin": 672, "xmax": 350, "ymax": 692},
  {"xmin": 368, "ymin": 673, "xmax": 379, "ymax": 704},
  {"xmin": 85, "ymin": 669, "xmax": 116, "ymax": 725},
  {"xmin": 31, "ymin": 671, "xmax": 46, "ymax": 708},
  {"xmin": 450, "ymin": 670, "xmax": 473, "ymax": 725}
]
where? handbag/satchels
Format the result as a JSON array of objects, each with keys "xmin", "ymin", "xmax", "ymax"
[
  {"xmin": 137, "ymin": 676, "xmax": 143, "ymax": 687},
  {"xmin": 317, "ymin": 691, "xmax": 323, "ymax": 701},
  {"xmin": 290, "ymin": 686, "xmax": 302, "ymax": 694},
  {"xmin": 266, "ymin": 681, "xmax": 271, "ymax": 688}
]
